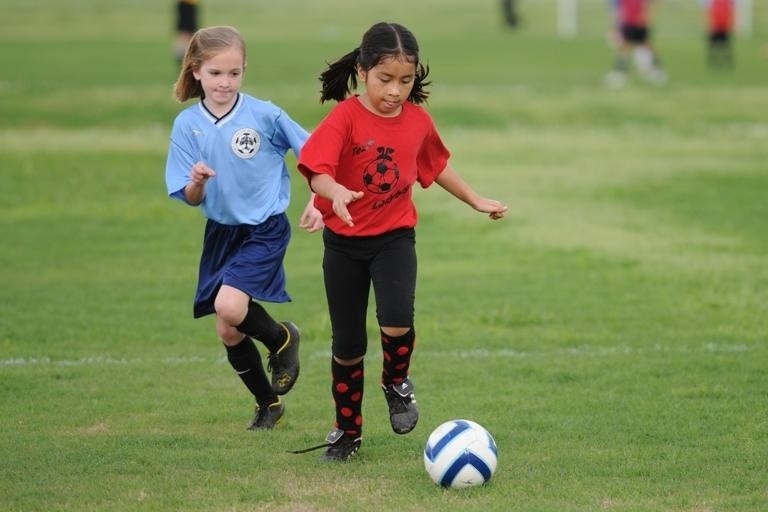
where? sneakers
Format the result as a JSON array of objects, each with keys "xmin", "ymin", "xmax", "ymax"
[
  {"xmin": 270, "ymin": 320, "xmax": 301, "ymax": 395},
  {"xmin": 381, "ymin": 378, "xmax": 419, "ymax": 435},
  {"xmin": 246, "ymin": 395, "xmax": 285, "ymax": 430},
  {"xmin": 319, "ymin": 429, "xmax": 362, "ymax": 461}
]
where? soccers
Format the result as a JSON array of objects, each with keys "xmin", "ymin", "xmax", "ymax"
[{"xmin": 423, "ymin": 418, "xmax": 499, "ymax": 489}]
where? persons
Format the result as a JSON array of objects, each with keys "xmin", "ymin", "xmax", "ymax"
[
  {"xmin": 503, "ymin": 0, "xmax": 753, "ymax": 88},
  {"xmin": 165, "ymin": 26, "xmax": 325, "ymax": 430},
  {"xmin": 298, "ymin": 22, "xmax": 509, "ymax": 459},
  {"xmin": 170, "ymin": 0, "xmax": 200, "ymax": 70}
]
